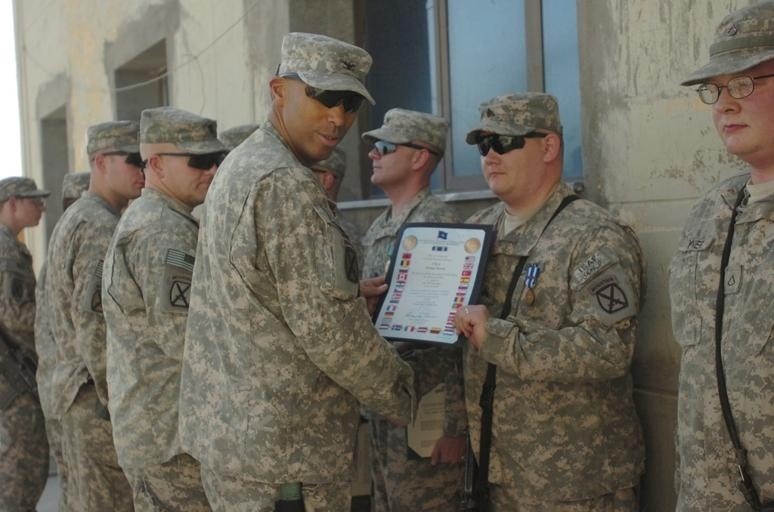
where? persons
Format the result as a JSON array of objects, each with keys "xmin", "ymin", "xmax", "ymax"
[{"xmin": 667, "ymin": 0, "xmax": 774, "ymax": 512}]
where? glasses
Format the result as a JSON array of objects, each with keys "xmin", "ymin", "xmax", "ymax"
[
  {"xmin": 373, "ymin": 140, "xmax": 439, "ymax": 157},
  {"xmin": 305, "ymin": 87, "xmax": 363, "ymax": 112},
  {"xmin": 126, "ymin": 153, "xmax": 145, "ymax": 168},
  {"xmin": 188, "ymin": 152, "xmax": 228, "ymax": 170},
  {"xmin": 478, "ymin": 132, "xmax": 547, "ymax": 156},
  {"xmin": 697, "ymin": 74, "xmax": 774, "ymax": 105}
]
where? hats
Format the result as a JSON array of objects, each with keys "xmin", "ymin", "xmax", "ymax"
[
  {"xmin": 361, "ymin": 108, "xmax": 448, "ymax": 157},
  {"xmin": 300, "ymin": 145, "xmax": 346, "ymax": 178},
  {"xmin": 275, "ymin": 32, "xmax": 376, "ymax": 107},
  {"xmin": 465, "ymin": 92, "xmax": 563, "ymax": 145},
  {"xmin": 61, "ymin": 172, "xmax": 90, "ymax": 199},
  {"xmin": 220, "ymin": 125, "xmax": 259, "ymax": 150},
  {"xmin": 0, "ymin": 177, "xmax": 50, "ymax": 203},
  {"xmin": 139, "ymin": 106, "xmax": 230, "ymax": 162},
  {"xmin": 680, "ymin": 0, "xmax": 774, "ymax": 85},
  {"xmin": 87, "ymin": 120, "xmax": 139, "ymax": 160}
]
